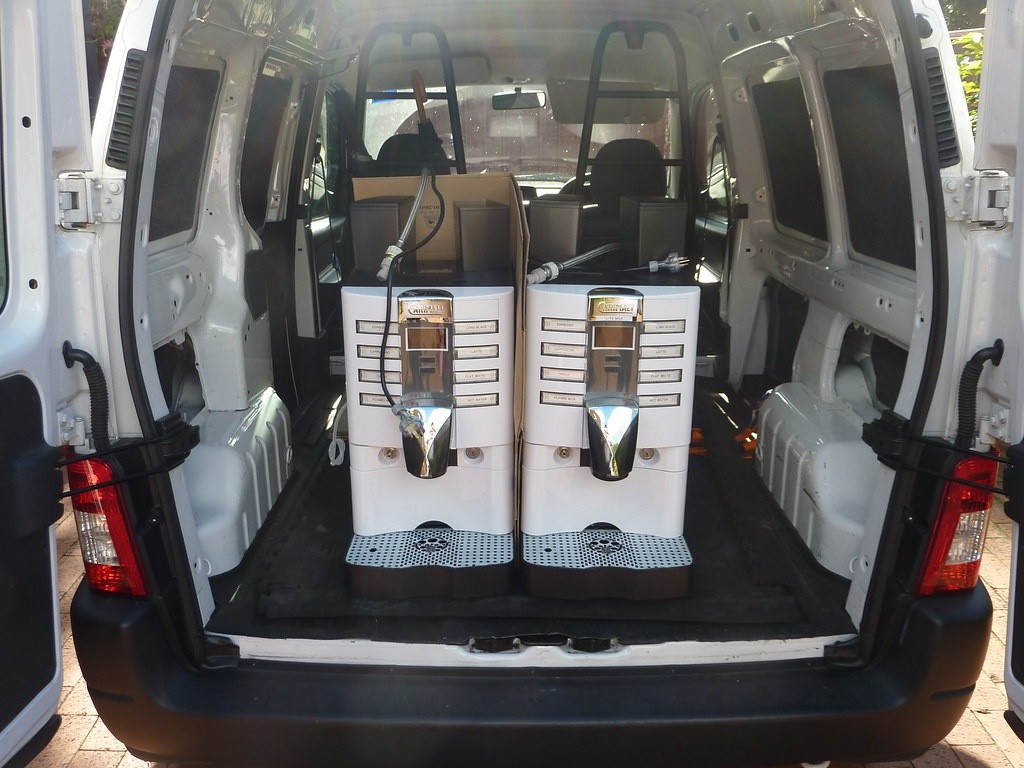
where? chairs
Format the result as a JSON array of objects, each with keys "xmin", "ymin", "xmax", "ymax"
[
  {"xmin": 372, "ymin": 133, "xmax": 450, "ymax": 176},
  {"xmin": 580, "ymin": 138, "xmax": 666, "ymax": 267}
]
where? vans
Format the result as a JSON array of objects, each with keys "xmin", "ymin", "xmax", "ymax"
[{"xmin": 0, "ymin": 0, "xmax": 1024, "ymax": 768}]
